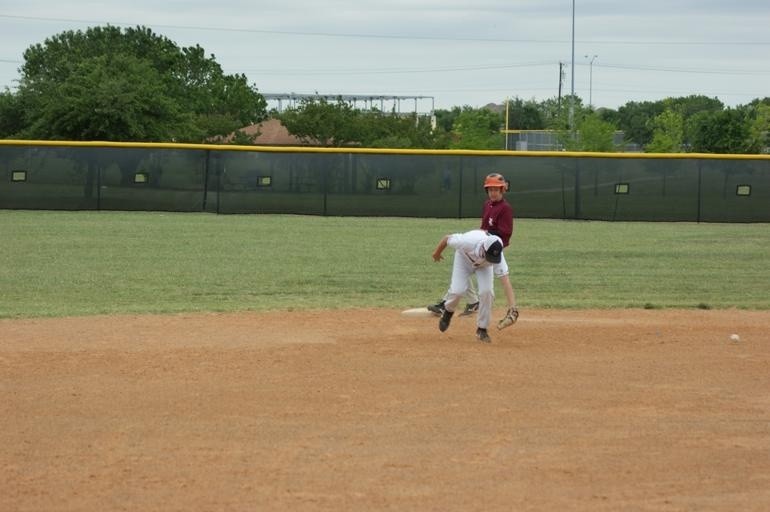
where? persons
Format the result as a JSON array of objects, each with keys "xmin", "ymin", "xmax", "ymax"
[
  {"xmin": 431, "ymin": 228, "xmax": 519, "ymax": 343},
  {"xmin": 426, "ymin": 172, "xmax": 513, "ymax": 317}
]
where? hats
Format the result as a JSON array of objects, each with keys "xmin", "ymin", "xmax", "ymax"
[{"xmin": 483, "ymin": 235, "xmax": 504, "ymax": 265}]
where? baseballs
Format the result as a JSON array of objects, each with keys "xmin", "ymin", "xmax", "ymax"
[{"xmin": 730, "ymin": 334, "xmax": 739, "ymax": 343}]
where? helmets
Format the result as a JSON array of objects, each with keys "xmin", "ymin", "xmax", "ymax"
[{"xmin": 482, "ymin": 173, "xmax": 510, "ymax": 193}]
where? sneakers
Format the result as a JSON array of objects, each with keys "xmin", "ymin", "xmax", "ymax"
[
  {"xmin": 456, "ymin": 300, "xmax": 480, "ymax": 317},
  {"xmin": 427, "ymin": 300, "xmax": 446, "ymax": 312},
  {"xmin": 474, "ymin": 326, "xmax": 492, "ymax": 344},
  {"xmin": 438, "ymin": 310, "xmax": 454, "ymax": 333}
]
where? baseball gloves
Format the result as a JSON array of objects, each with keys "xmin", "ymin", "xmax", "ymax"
[{"xmin": 497, "ymin": 307, "xmax": 519, "ymax": 329}]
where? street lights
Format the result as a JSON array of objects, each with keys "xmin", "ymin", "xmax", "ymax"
[{"xmin": 584, "ymin": 54, "xmax": 599, "ymax": 108}]
